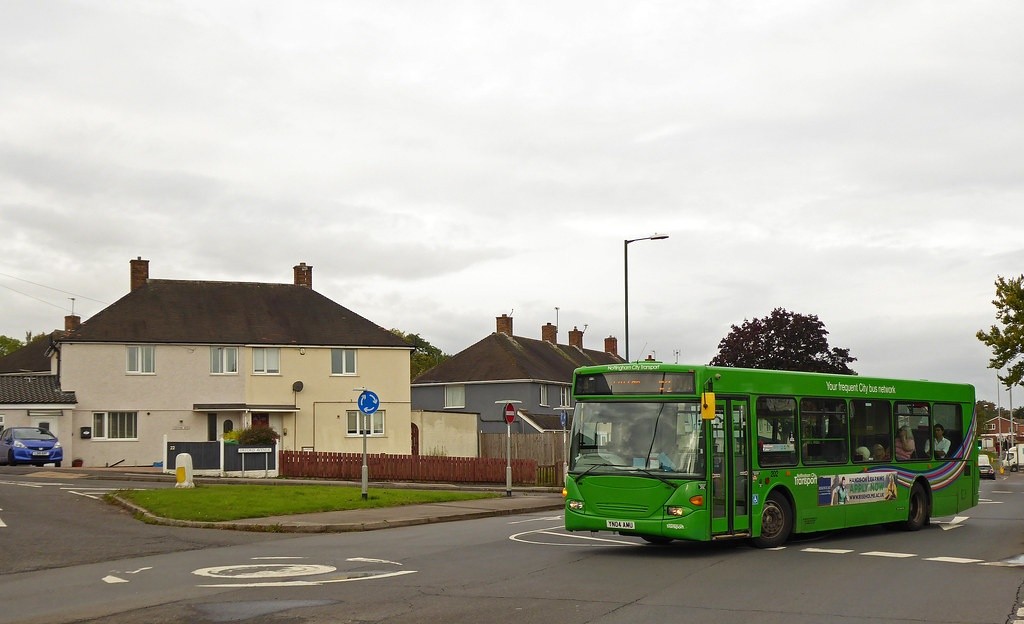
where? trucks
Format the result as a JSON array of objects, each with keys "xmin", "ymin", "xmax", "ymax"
[{"xmin": 1002, "ymin": 443, "xmax": 1024, "ymax": 472}]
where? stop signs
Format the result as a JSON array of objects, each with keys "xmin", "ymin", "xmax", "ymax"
[{"xmin": 505, "ymin": 403, "xmax": 515, "ymax": 425}]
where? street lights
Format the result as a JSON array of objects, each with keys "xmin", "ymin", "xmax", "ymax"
[
  {"xmin": 624, "ymin": 232, "xmax": 670, "ymax": 363},
  {"xmin": 1007, "ymin": 366, "xmax": 1014, "ymax": 447}
]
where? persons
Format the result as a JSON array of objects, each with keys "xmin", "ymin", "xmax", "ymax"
[
  {"xmin": 605, "ymin": 421, "xmax": 640, "ymax": 455},
  {"xmin": 802, "ymin": 423, "xmax": 951, "ymax": 461}
]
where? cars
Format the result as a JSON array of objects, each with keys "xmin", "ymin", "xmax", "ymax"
[
  {"xmin": 979, "ymin": 453, "xmax": 995, "ymax": 479},
  {"xmin": 0, "ymin": 427, "xmax": 64, "ymax": 467}
]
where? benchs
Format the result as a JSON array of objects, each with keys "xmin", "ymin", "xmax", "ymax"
[{"xmin": 897, "ymin": 430, "xmax": 964, "ymax": 458}]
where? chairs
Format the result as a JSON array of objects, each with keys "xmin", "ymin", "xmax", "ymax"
[{"xmin": 824, "ymin": 435, "xmax": 917, "ymax": 463}]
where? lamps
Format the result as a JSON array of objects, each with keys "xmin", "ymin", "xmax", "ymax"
[
  {"xmin": 298, "ymin": 348, "xmax": 306, "ymax": 355},
  {"xmin": 282, "ymin": 428, "xmax": 287, "ymax": 436}
]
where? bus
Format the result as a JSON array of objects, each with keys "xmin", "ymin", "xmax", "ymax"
[{"xmin": 561, "ymin": 364, "xmax": 979, "ymax": 550}]
